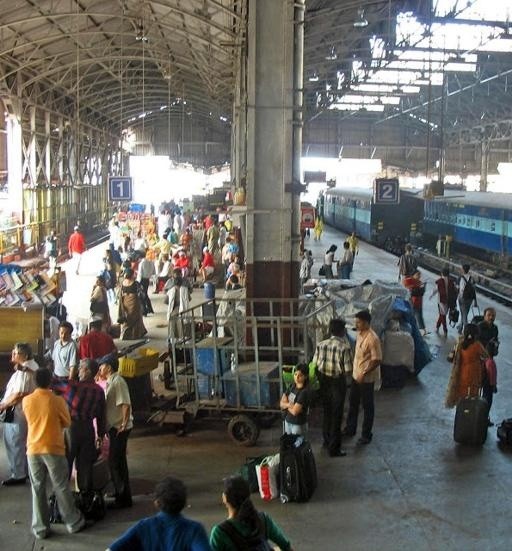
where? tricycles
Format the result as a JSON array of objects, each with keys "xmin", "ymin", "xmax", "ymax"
[{"xmin": 113, "ymin": 298, "xmax": 338, "ymax": 447}]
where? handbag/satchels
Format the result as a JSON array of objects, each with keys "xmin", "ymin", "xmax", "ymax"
[
  {"xmin": 90, "ymin": 442, "xmax": 112, "ymax": 491},
  {"xmin": 281, "ymin": 362, "xmax": 320, "ymax": 392},
  {"xmin": 48, "ymin": 490, "xmax": 107, "ymax": 524},
  {"xmin": 448, "ymin": 308, "xmax": 460, "ymax": 328},
  {"xmin": 497, "ymin": 418, "xmax": 512, "ymax": 451},
  {"xmin": 90, "ymin": 289, "xmax": 107, "ymax": 313},
  {"xmin": 0, "ymin": 403, "xmax": 15, "ymax": 423},
  {"xmin": 240, "ymin": 454, "xmax": 266, "ymax": 494},
  {"xmin": 319, "ymin": 265, "xmax": 326, "ymax": 276},
  {"xmin": 256, "ymin": 455, "xmax": 279, "ymax": 503},
  {"xmin": 412, "ymin": 283, "xmax": 427, "ymax": 297}
]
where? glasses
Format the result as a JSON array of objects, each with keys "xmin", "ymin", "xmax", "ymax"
[{"xmin": 12, "ymin": 350, "xmax": 24, "ymax": 355}]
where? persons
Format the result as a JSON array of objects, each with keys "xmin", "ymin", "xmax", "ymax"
[
  {"xmin": 314, "ymin": 215, "xmax": 323, "ymax": 241},
  {"xmin": 446, "ymin": 323, "xmax": 498, "ymax": 431},
  {"xmin": 149, "ymin": 199, "xmax": 194, "ymax": 226},
  {"xmin": 0, "ymin": 342, "xmax": 40, "ymax": 486},
  {"xmin": 403, "ymin": 270, "xmax": 426, "ymax": 337},
  {"xmin": 97, "ymin": 353, "xmax": 134, "ymax": 510},
  {"xmin": 10, "ymin": 358, "xmax": 107, "ymax": 516},
  {"xmin": 428, "ymin": 268, "xmax": 457, "ymax": 337},
  {"xmin": 68, "ymin": 225, "xmax": 86, "ymax": 275},
  {"xmin": 278, "ymin": 362, "xmax": 309, "ymax": 440},
  {"xmin": 104, "ymin": 475, "xmax": 210, "ymax": 551},
  {"xmin": 341, "ymin": 310, "xmax": 383, "ymax": 446},
  {"xmin": 194, "ymin": 214, "xmax": 244, "ymax": 290},
  {"xmin": 396, "ymin": 243, "xmax": 417, "ymax": 282},
  {"xmin": 164, "ymin": 276, "xmax": 192, "ymax": 362},
  {"xmin": 346, "ymin": 231, "xmax": 359, "ymax": 264},
  {"xmin": 44, "ymin": 321, "xmax": 77, "ymax": 385},
  {"xmin": 299, "ymin": 249, "xmax": 313, "ymax": 293},
  {"xmin": 455, "ymin": 264, "xmax": 477, "ymax": 334},
  {"xmin": 465, "ymin": 307, "xmax": 499, "ymax": 361},
  {"xmin": 78, "ymin": 316, "xmax": 117, "ymax": 358},
  {"xmin": 90, "ymin": 275, "xmax": 112, "ymax": 328},
  {"xmin": 22, "ymin": 367, "xmax": 85, "ymax": 539},
  {"xmin": 103, "ymin": 215, "xmax": 135, "ymax": 276},
  {"xmin": 339, "ymin": 241, "xmax": 353, "ymax": 280},
  {"xmin": 136, "ymin": 251, "xmax": 154, "ymax": 317},
  {"xmin": 324, "ymin": 245, "xmax": 338, "ymax": 280},
  {"xmin": 115, "ymin": 268, "xmax": 151, "ymax": 341},
  {"xmin": 314, "ymin": 316, "xmax": 348, "ymax": 456},
  {"xmin": 210, "ymin": 475, "xmax": 296, "ymax": 551},
  {"xmin": 41, "ymin": 230, "xmax": 61, "ymax": 279},
  {"xmin": 154, "ymin": 226, "xmax": 194, "ymax": 293}
]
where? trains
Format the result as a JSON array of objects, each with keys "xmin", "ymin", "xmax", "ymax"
[
  {"xmin": 400, "ymin": 188, "xmax": 512, "ymax": 270},
  {"xmin": 426, "ymin": 191, "xmax": 511, "ymax": 263},
  {"xmin": 320, "ymin": 186, "xmax": 411, "ymax": 251},
  {"xmin": 322, "ymin": 186, "xmax": 426, "ymax": 247}
]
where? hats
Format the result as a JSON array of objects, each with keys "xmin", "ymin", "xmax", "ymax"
[
  {"xmin": 122, "ymin": 278, "xmax": 135, "ymax": 287},
  {"xmin": 98, "ymin": 352, "xmax": 119, "ymax": 371}
]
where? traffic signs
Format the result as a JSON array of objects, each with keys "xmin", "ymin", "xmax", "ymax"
[{"xmin": 302, "ymin": 208, "xmax": 315, "ymax": 228}]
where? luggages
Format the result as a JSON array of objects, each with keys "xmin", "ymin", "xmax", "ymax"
[
  {"xmin": 453, "ymin": 383, "xmax": 489, "ymax": 445},
  {"xmin": 471, "ymin": 305, "xmax": 484, "ymax": 325},
  {"xmin": 280, "ymin": 410, "xmax": 318, "ymax": 505}
]
